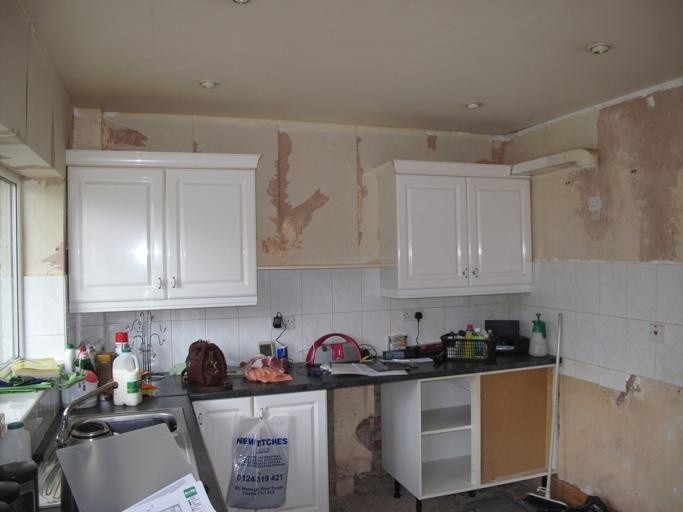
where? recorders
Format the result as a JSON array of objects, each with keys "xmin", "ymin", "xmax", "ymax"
[{"xmin": 306, "ymin": 333, "xmax": 363, "ymax": 366}]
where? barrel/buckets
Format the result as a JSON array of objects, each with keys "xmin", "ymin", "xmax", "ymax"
[{"xmin": 112, "ymin": 344, "xmax": 143, "ymax": 407}]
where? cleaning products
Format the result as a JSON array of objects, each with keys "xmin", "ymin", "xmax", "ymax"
[{"xmin": 529, "ymin": 312, "xmax": 549, "ymax": 357}]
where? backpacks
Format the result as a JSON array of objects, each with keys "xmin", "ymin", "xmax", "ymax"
[{"xmin": 181, "ymin": 338, "xmax": 229, "ymax": 388}]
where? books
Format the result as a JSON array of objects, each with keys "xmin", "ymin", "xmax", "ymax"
[
  {"xmin": 352, "ymin": 362, "xmax": 408, "ymax": 378},
  {"xmin": 9, "ymin": 355, "xmax": 59, "ymax": 379},
  {"xmin": 330, "ymin": 362, "xmax": 363, "ymax": 376},
  {"xmin": 409, "ymin": 361, "xmax": 437, "ymax": 369}
]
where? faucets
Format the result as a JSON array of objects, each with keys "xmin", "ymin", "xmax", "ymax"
[{"xmin": 54, "ymin": 381, "xmax": 118, "ymax": 446}]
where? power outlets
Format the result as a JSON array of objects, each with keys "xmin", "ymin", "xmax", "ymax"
[
  {"xmin": 400, "ymin": 310, "xmax": 424, "ymax": 321},
  {"xmin": 270, "ymin": 313, "xmax": 296, "ymax": 330}
]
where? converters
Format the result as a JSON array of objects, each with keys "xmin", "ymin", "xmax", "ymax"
[{"xmin": 273, "ymin": 317, "xmax": 282, "ymax": 328}]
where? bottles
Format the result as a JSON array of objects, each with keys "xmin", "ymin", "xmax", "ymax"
[
  {"xmin": 114, "ymin": 332, "xmax": 128, "ymax": 354},
  {"xmin": 447, "ymin": 324, "xmax": 494, "ymax": 359}
]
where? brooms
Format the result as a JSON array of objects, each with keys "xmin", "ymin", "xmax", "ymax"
[{"xmin": 524, "ymin": 312, "xmax": 567, "ymax": 511}]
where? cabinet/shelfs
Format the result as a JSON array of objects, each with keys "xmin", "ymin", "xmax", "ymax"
[
  {"xmin": 374, "ymin": 159, "xmax": 533, "ymax": 298},
  {"xmin": 380, "ymin": 363, "xmax": 556, "ymax": 512},
  {"xmin": 64, "ymin": 148, "xmax": 261, "ymax": 315},
  {"xmin": 192, "ymin": 390, "xmax": 330, "ymax": 512}
]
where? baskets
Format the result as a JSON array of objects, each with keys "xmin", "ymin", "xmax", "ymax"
[{"xmin": 438, "ymin": 328, "xmax": 494, "ymax": 363}]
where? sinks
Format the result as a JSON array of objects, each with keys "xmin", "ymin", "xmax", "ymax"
[{"xmin": 69, "ymin": 406, "xmax": 187, "ymax": 435}]
